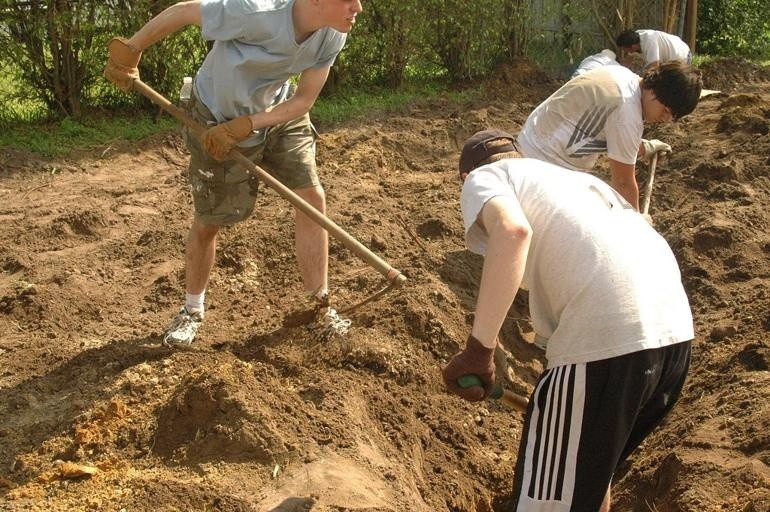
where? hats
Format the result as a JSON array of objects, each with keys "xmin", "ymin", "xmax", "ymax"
[{"xmin": 459, "ymin": 129, "xmax": 518, "ymax": 178}]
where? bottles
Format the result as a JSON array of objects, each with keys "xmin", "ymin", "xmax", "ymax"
[{"xmin": 180, "ymin": 75, "xmax": 192, "ymax": 109}]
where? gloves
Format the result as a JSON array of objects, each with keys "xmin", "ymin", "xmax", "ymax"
[
  {"xmin": 443, "ymin": 334, "xmax": 496, "ymax": 402},
  {"xmin": 201, "ymin": 112, "xmax": 254, "ymax": 162},
  {"xmin": 105, "ymin": 38, "xmax": 139, "ymax": 94},
  {"xmin": 638, "ymin": 139, "xmax": 672, "ymax": 166}
]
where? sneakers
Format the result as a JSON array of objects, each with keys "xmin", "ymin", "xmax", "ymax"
[
  {"xmin": 313, "ymin": 303, "xmax": 351, "ymax": 338},
  {"xmin": 165, "ymin": 305, "xmax": 204, "ymax": 347}
]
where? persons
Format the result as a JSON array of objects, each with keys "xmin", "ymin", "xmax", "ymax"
[
  {"xmin": 615, "ymin": 27, "xmax": 695, "ymax": 77},
  {"xmin": 440, "ymin": 129, "xmax": 696, "ymax": 512},
  {"xmin": 100, "ymin": 0, "xmax": 365, "ymax": 348},
  {"xmin": 515, "ymin": 56, "xmax": 704, "ymax": 352},
  {"xmin": 572, "ymin": 47, "xmax": 619, "ymax": 77}
]
